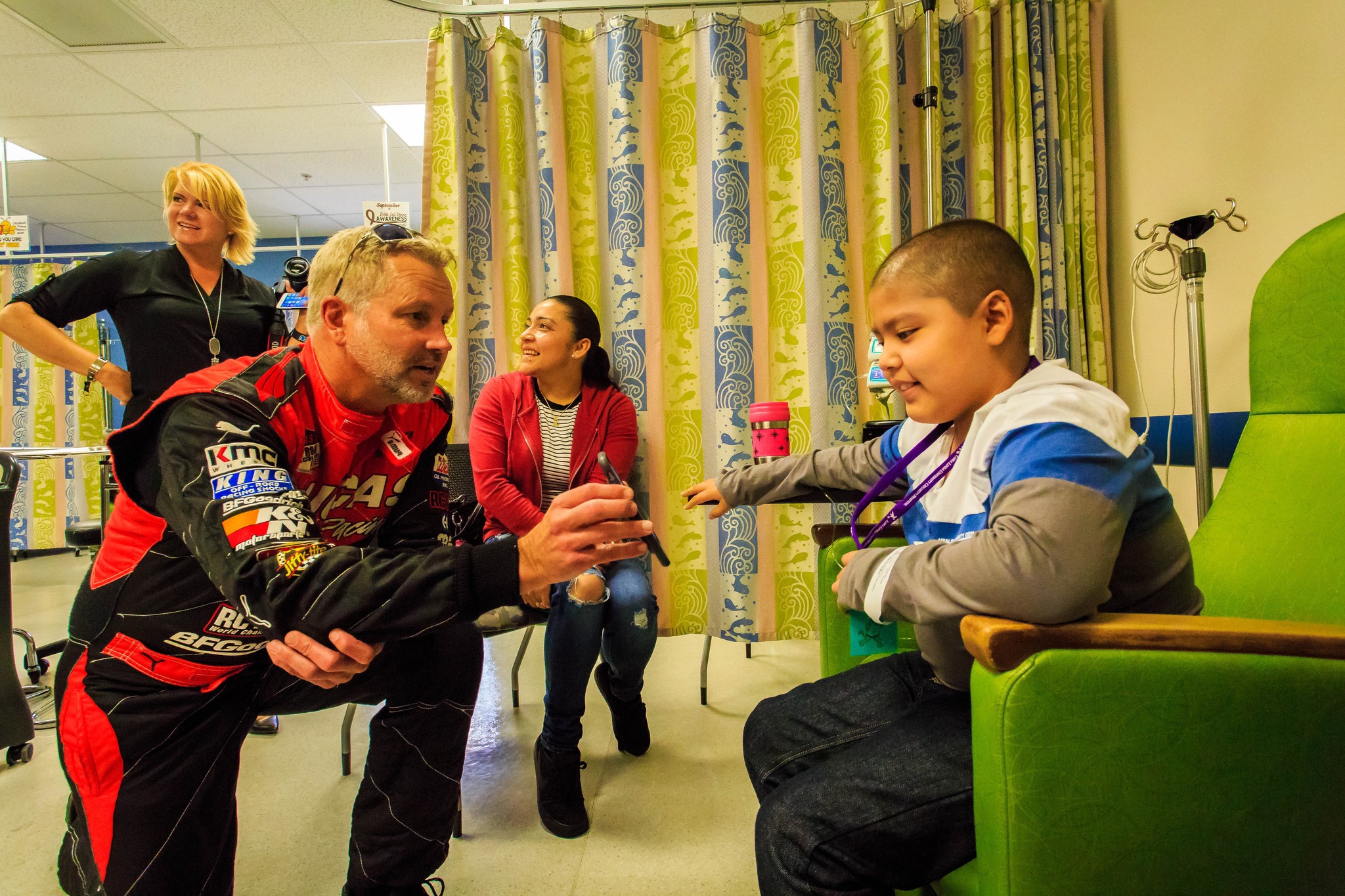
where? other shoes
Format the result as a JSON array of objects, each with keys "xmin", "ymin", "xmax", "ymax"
[
  {"xmin": 57, "ymin": 791, "xmax": 86, "ymax": 896},
  {"xmin": 341, "ymin": 876, "xmax": 445, "ymax": 896}
]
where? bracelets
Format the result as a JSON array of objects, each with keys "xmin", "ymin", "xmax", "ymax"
[
  {"xmin": 291, "ymin": 327, "xmax": 309, "ymax": 343},
  {"xmin": 83, "ymin": 356, "xmax": 108, "ymax": 396}
]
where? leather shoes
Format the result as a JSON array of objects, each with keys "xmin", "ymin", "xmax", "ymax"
[{"xmin": 249, "ymin": 715, "xmax": 279, "ymax": 734}]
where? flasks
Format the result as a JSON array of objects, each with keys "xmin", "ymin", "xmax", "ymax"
[{"xmin": 750, "ymin": 401, "xmax": 791, "ymax": 467}]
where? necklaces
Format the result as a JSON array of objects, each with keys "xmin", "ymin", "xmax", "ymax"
[
  {"xmin": 190, "ymin": 257, "xmax": 224, "ymax": 367},
  {"xmin": 538, "ymin": 388, "xmax": 582, "ymax": 427}
]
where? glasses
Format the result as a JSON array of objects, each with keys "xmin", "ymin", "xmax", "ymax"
[{"xmin": 332, "ymin": 223, "xmax": 429, "ymax": 299}]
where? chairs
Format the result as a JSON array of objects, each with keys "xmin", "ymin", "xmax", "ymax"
[{"xmin": 811, "ymin": 213, "xmax": 1345, "ymax": 896}]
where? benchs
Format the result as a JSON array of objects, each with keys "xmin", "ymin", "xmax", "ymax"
[{"xmin": 340, "ymin": 444, "xmax": 554, "ymax": 838}]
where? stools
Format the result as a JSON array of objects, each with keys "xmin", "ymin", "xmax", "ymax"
[{"xmin": 24, "ymin": 517, "xmax": 102, "ymax": 675}]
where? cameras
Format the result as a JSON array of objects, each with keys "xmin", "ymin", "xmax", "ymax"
[{"xmin": 273, "ymin": 256, "xmax": 311, "ymax": 300}]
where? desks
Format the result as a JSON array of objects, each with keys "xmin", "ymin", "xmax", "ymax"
[{"xmin": 688, "ymin": 494, "xmax": 909, "ymax": 504}]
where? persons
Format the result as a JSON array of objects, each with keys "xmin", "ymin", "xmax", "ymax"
[
  {"xmin": 0, "ymin": 163, "xmax": 307, "ymax": 734},
  {"xmin": 54, "ymin": 221, "xmax": 652, "ymax": 896},
  {"xmin": 466, "ymin": 296, "xmax": 660, "ymax": 839},
  {"xmin": 681, "ymin": 216, "xmax": 1206, "ymax": 894}
]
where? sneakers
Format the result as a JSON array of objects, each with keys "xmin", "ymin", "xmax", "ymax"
[
  {"xmin": 534, "ymin": 732, "xmax": 590, "ymax": 838},
  {"xmin": 593, "ymin": 660, "xmax": 651, "ymax": 756}
]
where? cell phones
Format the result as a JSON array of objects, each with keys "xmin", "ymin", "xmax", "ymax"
[
  {"xmin": 598, "ymin": 452, "xmax": 671, "ymax": 567},
  {"xmin": 276, "ymin": 293, "xmax": 309, "ymax": 310}
]
